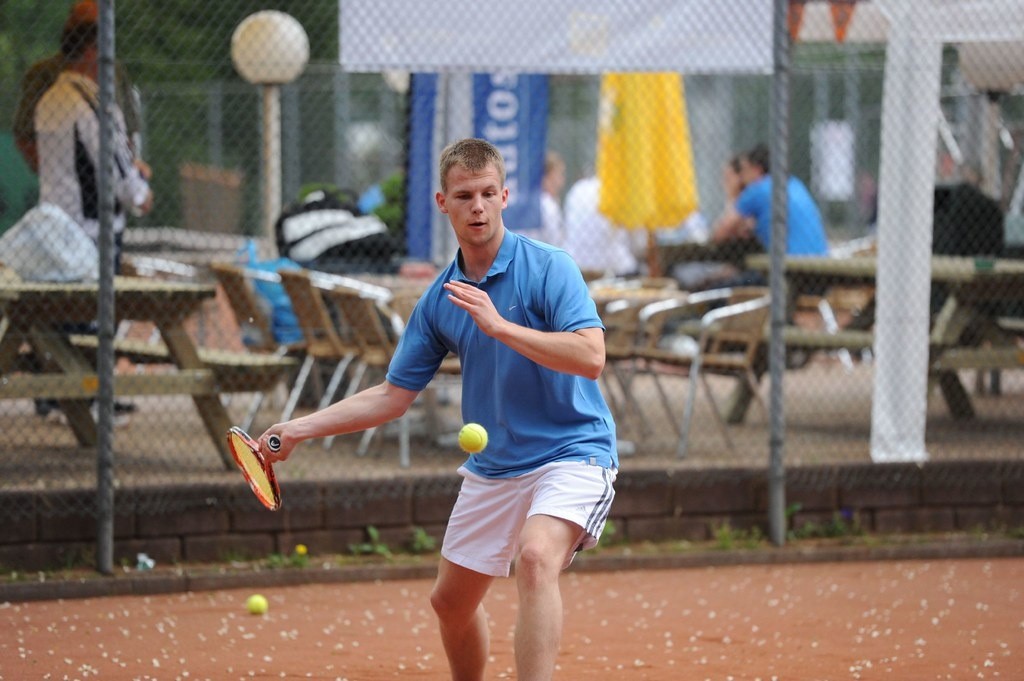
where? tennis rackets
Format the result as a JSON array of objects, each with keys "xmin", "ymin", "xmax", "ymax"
[{"xmin": 226, "ymin": 426, "xmax": 283, "ymax": 512}]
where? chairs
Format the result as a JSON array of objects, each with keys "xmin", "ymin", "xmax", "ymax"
[{"xmin": 0, "ymin": 198, "xmax": 1024, "ymax": 472}]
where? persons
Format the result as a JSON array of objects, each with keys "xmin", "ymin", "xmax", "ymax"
[
  {"xmin": 513, "ymin": 147, "xmax": 562, "ymax": 246},
  {"xmin": 12, "ymin": 1, "xmax": 152, "ymax": 180},
  {"xmin": 34, "ymin": 21, "xmax": 153, "ymax": 427},
  {"xmin": 724, "ymin": 158, "xmax": 746, "ymax": 206},
  {"xmin": 257, "ymin": 138, "xmax": 619, "ymax": 679},
  {"xmin": 564, "ymin": 170, "xmax": 641, "ymax": 284},
  {"xmin": 714, "ymin": 142, "xmax": 831, "ymax": 295}
]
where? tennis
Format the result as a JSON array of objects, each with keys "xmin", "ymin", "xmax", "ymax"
[
  {"xmin": 246, "ymin": 594, "xmax": 268, "ymax": 615},
  {"xmin": 458, "ymin": 422, "xmax": 487, "ymax": 454}
]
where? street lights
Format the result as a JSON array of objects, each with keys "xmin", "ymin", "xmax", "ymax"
[{"xmin": 230, "ymin": 11, "xmax": 311, "ymax": 254}]
